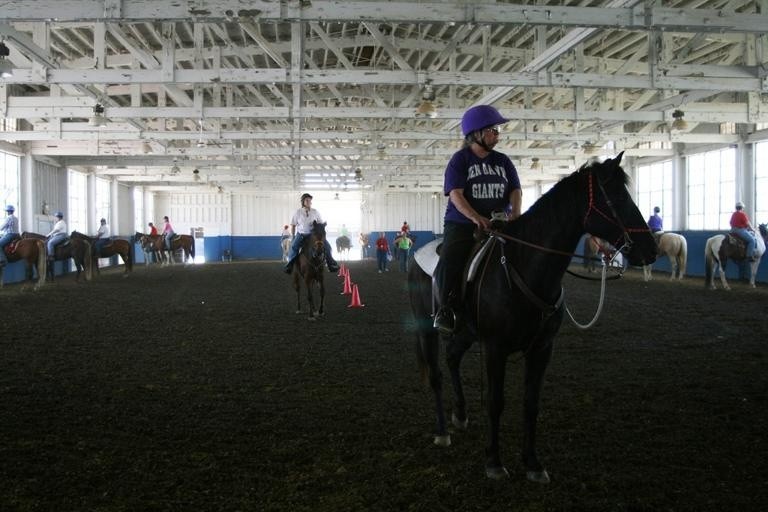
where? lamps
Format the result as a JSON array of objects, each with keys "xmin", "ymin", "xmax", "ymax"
[
  {"xmin": 530, "ymin": 158, "xmax": 540, "ymax": 170},
  {"xmin": 354, "ymin": 169, "xmax": 365, "ymax": 181},
  {"xmin": 672, "ymin": 110, "xmax": 688, "ymax": 131},
  {"xmin": 0, "ymin": 42, "xmax": 224, "ymax": 195},
  {"xmin": 417, "ymin": 83, "xmax": 437, "ymax": 117}
]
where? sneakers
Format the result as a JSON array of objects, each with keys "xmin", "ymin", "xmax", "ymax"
[{"xmin": 378, "ymin": 267, "xmax": 390, "ymax": 274}]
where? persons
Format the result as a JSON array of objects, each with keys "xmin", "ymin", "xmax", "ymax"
[
  {"xmin": 648, "ymin": 206, "xmax": 663, "ymax": 237},
  {"xmin": 288, "ymin": 194, "xmax": 340, "ymax": 271},
  {"xmin": 432, "ymin": 105, "xmax": 522, "ymax": 330},
  {"xmin": 161, "ymin": 216, "xmax": 174, "ymax": 250},
  {"xmin": 94, "ymin": 218, "xmax": 109, "ymax": 258},
  {"xmin": 0, "ymin": 205, "xmax": 20, "ymax": 268},
  {"xmin": 148, "ymin": 222, "xmax": 157, "ymax": 247},
  {"xmin": 46, "ymin": 211, "xmax": 67, "ymax": 261},
  {"xmin": 729, "ymin": 203, "xmax": 757, "ymax": 263},
  {"xmin": 357, "ymin": 222, "xmax": 413, "ymax": 273},
  {"xmin": 341, "ymin": 223, "xmax": 347, "ymax": 238},
  {"xmin": 281, "ymin": 225, "xmax": 289, "ymax": 239}
]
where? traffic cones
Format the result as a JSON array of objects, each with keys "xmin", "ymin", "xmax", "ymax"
[
  {"xmin": 339, "ymin": 268, "xmax": 354, "ymax": 296},
  {"xmin": 338, "ymin": 262, "xmax": 347, "ymax": 277},
  {"xmin": 347, "ymin": 283, "xmax": 366, "ymax": 308}
]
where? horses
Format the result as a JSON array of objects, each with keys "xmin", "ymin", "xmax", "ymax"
[
  {"xmin": 140, "ymin": 234, "xmax": 195, "ymax": 269},
  {"xmin": 0, "ymin": 237, "xmax": 47, "ymax": 292},
  {"xmin": 70, "ymin": 231, "xmax": 133, "ymax": 281},
  {"xmin": 133, "ymin": 232, "xmax": 173, "ymax": 265},
  {"xmin": 281, "ymin": 232, "xmax": 291, "ymax": 263},
  {"xmin": 282, "ymin": 219, "xmax": 327, "ymax": 322},
  {"xmin": 702, "ymin": 224, "xmax": 768, "ymax": 292},
  {"xmin": 641, "ymin": 231, "xmax": 686, "ymax": 283},
  {"xmin": 583, "ymin": 235, "xmax": 619, "ymax": 274},
  {"xmin": 21, "ymin": 231, "xmax": 91, "ymax": 283},
  {"xmin": 405, "ymin": 149, "xmax": 659, "ymax": 484}
]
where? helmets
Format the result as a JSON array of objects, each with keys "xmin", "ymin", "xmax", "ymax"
[
  {"xmin": 462, "ymin": 104, "xmax": 509, "ymax": 137},
  {"xmin": 5, "ymin": 205, "xmax": 15, "ymax": 211},
  {"xmin": 734, "ymin": 202, "xmax": 746, "ymax": 209},
  {"xmin": 301, "ymin": 193, "xmax": 312, "ymax": 200},
  {"xmin": 55, "ymin": 211, "xmax": 64, "ymax": 217}
]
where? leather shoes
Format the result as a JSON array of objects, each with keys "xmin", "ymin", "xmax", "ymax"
[{"xmin": 431, "ymin": 305, "xmax": 458, "ymax": 335}]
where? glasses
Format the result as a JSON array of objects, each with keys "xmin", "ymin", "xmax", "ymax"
[{"xmin": 481, "ymin": 124, "xmax": 502, "ymax": 136}]
